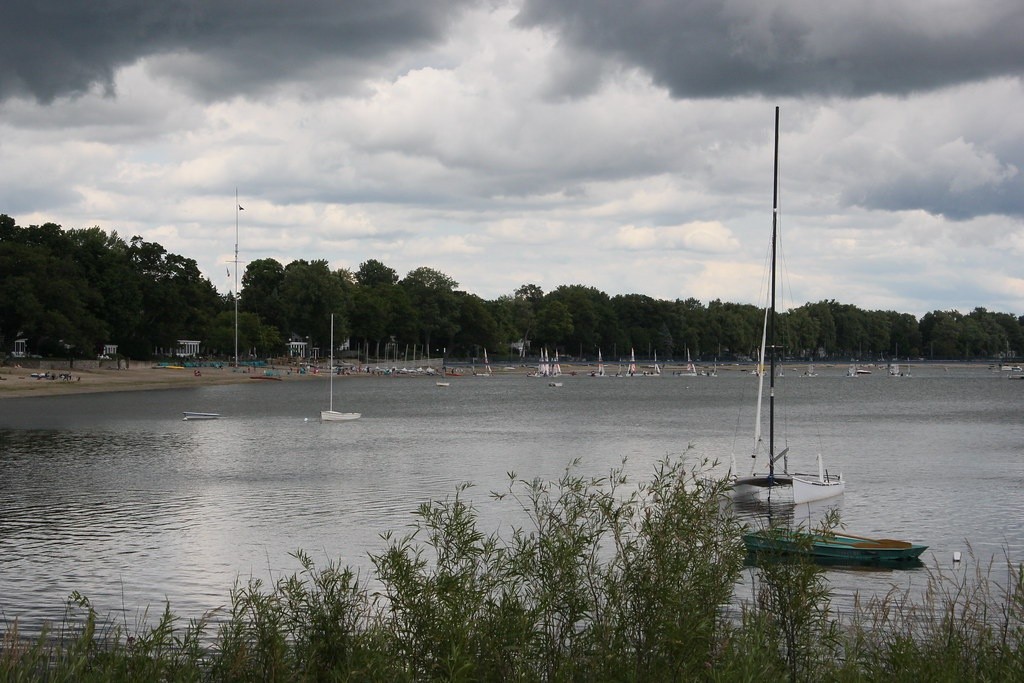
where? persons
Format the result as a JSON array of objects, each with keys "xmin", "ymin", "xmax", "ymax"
[
  {"xmin": 180, "ymin": 354, "xmax": 370, "ymax": 377},
  {"xmin": 36, "ymin": 370, "xmax": 71, "ymax": 382}
]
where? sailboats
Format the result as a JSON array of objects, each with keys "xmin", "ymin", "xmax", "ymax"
[
  {"xmin": 587, "ymin": 348, "xmax": 607, "ymax": 377},
  {"xmin": 678, "ymin": 347, "xmax": 700, "ymax": 377},
  {"xmin": 320, "ymin": 314, "xmax": 363, "ymax": 421},
  {"xmin": 721, "ymin": 105, "xmax": 847, "ymax": 508},
  {"xmin": 624, "ymin": 348, "xmax": 663, "ymax": 377},
  {"xmin": 526, "ymin": 346, "xmax": 565, "ymax": 378}
]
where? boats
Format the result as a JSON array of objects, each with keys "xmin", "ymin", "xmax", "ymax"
[
  {"xmin": 742, "ymin": 527, "xmax": 930, "ymax": 568},
  {"xmin": 181, "ymin": 410, "xmax": 222, "ymax": 421}
]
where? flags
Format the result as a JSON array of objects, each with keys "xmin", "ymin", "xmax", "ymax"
[
  {"xmin": 227, "ymin": 268, "xmax": 230, "ymax": 277},
  {"xmin": 239, "ymin": 206, "xmax": 244, "ymax": 211}
]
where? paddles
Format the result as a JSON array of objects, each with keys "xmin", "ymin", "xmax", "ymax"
[
  {"xmin": 812, "ymin": 528, "xmax": 912, "ymax": 548},
  {"xmin": 776, "ymin": 534, "xmax": 896, "ymax": 547}
]
